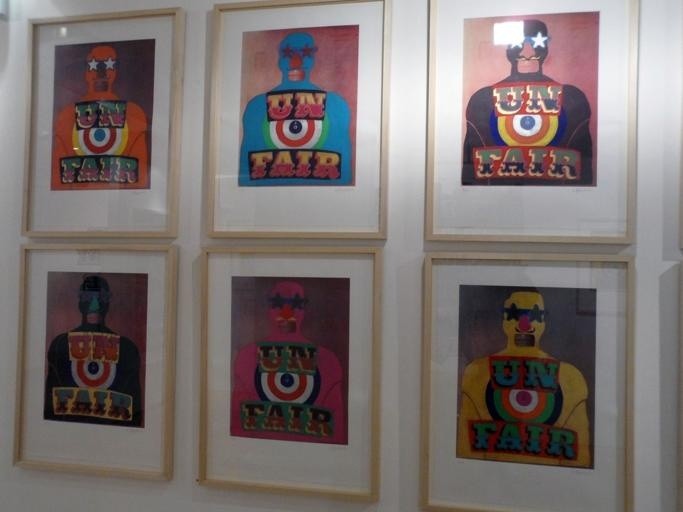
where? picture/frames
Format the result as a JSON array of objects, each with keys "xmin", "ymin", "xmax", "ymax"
[
  {"xmin": 14, "ymin": 239, "xmax": 179, "ymax": 481},
  {"xmin": 20, "ymin": 5, "xmax": 185, "ymax": 239},
  {"xmin": 207, "ymin": 2, "xmax": 392, "ymax": 242},
  {"xmin": 198, "ymin": 246, "xmax": 384, "ymax": 506},
  {"xmin": 423, "ymin": 0, "xmax": 642, "ymax": 248},
  {"xmin": 420, "ymin": 252, "xmax": 636, "ymax": 512}
]
